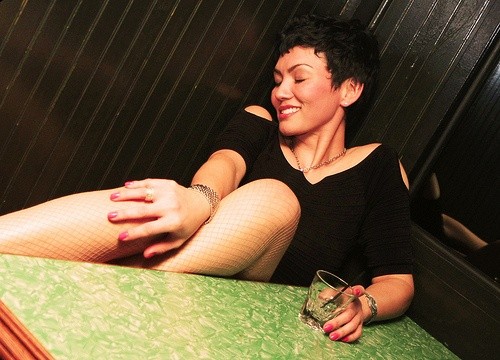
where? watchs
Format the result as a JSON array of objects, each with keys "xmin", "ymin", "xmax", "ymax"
[{"xmin": 364, "ymin": 291, "xmax": 377, "ymax": 326}]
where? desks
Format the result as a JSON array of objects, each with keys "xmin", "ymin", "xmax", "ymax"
[{"xmin": 0, "ymin": 252, "xmax": 460, "ymax": 360}]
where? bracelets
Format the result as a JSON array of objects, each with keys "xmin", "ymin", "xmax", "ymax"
[{"xmin": 190, "ymin": 181, "xmax": 218, "ymax": 226}]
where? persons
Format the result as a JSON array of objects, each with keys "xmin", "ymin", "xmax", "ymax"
[
  {"xmin": 0, "ymin": 16, "xmax": 418, "ymax": 345},
  {"xmin": 414, "ymin": 171, "xmax": 500, "ymax": 282}
]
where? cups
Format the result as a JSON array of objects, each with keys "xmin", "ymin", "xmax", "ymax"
[{"xmin": 298, "ymin": 271, "xmax": 354, "ymax": 331}]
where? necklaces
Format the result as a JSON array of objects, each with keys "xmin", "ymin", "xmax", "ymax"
[{"xmin": 288, "ymin": 148, "xmax": 347, "ymax": 173}]
